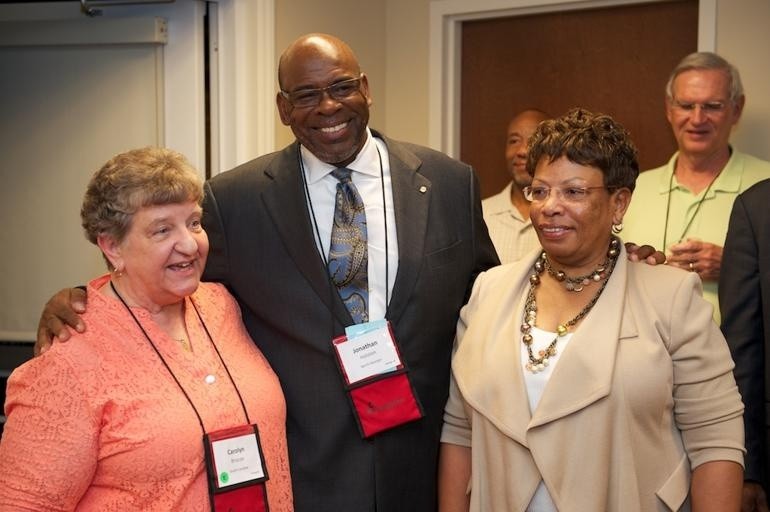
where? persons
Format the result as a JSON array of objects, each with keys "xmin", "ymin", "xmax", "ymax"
[
  {"xmin": 478, "ymin": 109, "xmax": 553, "ymax": 267},
  {"xmin": 610, "ymin": 52, "xmax": 769, "ymax": 328},
  {"xmin": 34, "ymin": 34, "xmax": 666, "ymax": 512},
  {"xmin": 717, "ymin": 178, "xmax": 770, "ymax": 512},
  {"xmin": 438, "ymin": 108, "xmax": 747, "ymax": 511},
  {"xmin": 0, "ymin": 146, "xmax": 293, "ymax": 512}
]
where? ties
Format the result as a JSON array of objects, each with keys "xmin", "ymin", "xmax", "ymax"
[{"xmin": 327, "ymin": 163, "xmax": 370, "ymax": 333}]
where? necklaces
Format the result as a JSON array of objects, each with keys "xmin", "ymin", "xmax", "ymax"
[
  {"xmin": 520, "ymin": 233, "xmax": 621, "ymax": 373},
  {"xmin": 115, "ymin": 287, "xmax": 191, "ymax": 350}
]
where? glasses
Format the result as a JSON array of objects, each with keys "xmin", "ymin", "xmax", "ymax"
[
  {"xmin": 669, "ymin": 99, "xmax": 731, "ymax": 112},
  {"xmin": 274, "ymin": 73, "xmax": 364, "ymax": 108},
  {"xmin": 519, "ymin": 182, "xmax": 621, "ymax": 203}
]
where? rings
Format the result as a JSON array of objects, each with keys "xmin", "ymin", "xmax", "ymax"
[{"xmin": 689, "ymin": 263, "xmax": 693, "ymax": 271}]
